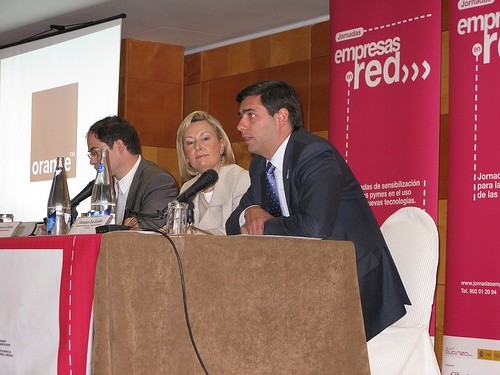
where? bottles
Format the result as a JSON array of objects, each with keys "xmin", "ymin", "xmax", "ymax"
[
  {"xmin": 90, "ymin": 148, "xmax": 116, "ymax": 224},
  {"xmin": 47, "ymin": 157, "xmax": 71, "ymax": 235}
]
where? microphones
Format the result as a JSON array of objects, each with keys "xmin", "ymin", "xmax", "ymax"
[
  {"xmin": 162, "ymin": 169, "xmax": 218, "ymax": 213},
  {"xmin": 43, "ymin": 179, "xmax": 96, "ymax": 225}
]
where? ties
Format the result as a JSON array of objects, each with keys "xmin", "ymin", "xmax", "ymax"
[
  {"xmin": 114, "ymin": 181, "xmax": 126, "ymax": 225},
  {"xmin": 262, "ymin": 162, "xmax": 283, "ymax": 218}
]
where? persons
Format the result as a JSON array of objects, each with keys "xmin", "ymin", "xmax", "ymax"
[
  {"xmin": 159, "ymin": 110, "xmax": 252, "ymax": 234},
  {"xmin": 40, "ymin": 116, "xmax": 178, "ymax": 235},
  {"xmin": 226, "ymin": 82, "xmax": 412, "ymax": 343}
]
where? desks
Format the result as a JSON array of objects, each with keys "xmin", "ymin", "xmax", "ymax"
[{"xmin": 0, "ymin": 232, "xmax": 372, "ymax": 375}]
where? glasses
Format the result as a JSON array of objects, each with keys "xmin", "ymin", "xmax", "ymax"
[{"xmin": 88, "ymin": 142, "xmax": 109, "ymax": 162}]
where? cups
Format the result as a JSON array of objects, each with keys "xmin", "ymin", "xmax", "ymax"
[
  {"xmin": 166, "ymin": 201, "xmax": 188, "ymax": 235},
  {"xmin": 0, "ymin": 214, "xmax": 13, "ymax": 223}
]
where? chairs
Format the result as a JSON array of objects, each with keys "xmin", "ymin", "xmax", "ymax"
[{"xmin": 364, "ymin": 206, "xmax": 443, "ymax": 375}]
